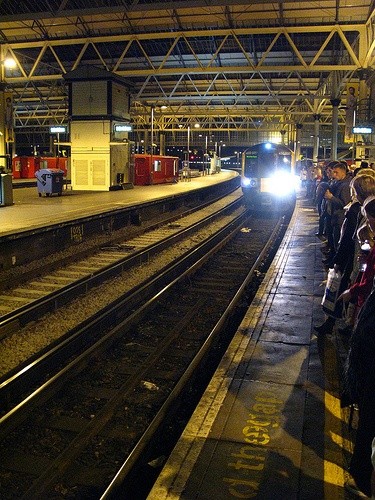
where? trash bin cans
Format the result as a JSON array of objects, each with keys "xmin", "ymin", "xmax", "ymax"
[
  {"xmin": 35, "ymin": 169, "xmax": 65, "ymax": 198},
  {"xmin": 1, "ymin": 173, "xmax": 15, "ymax": 207}
]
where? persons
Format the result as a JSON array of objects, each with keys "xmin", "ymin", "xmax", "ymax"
[{"xmin": 300, "ymin": 159, "xmax": 375, "ymax": 500}]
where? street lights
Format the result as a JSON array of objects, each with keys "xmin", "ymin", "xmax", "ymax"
[
  {"xmin": 187, "ymin": 124, "xmax": 200, "ymax": 182},
  {"xmin": 149, "ymin": 105, "xmax": 167, "ymax": 186}
]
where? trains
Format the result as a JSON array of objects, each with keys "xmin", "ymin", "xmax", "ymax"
[{"xmin": 240, "ymin": 142, "xmax": 296, "ymax": 213}]
